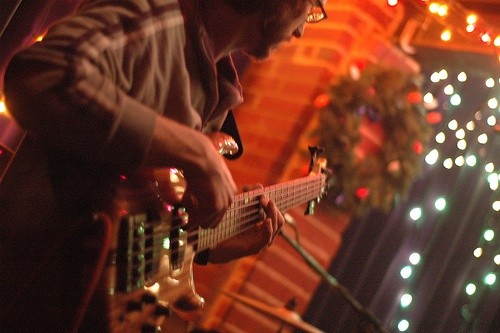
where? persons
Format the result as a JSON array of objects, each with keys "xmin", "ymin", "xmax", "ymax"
[{"xmin": 0, "ymin": 0, "xmax": 321, "ymax": 333}]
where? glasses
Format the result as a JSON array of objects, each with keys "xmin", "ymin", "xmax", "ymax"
[{"xmin": 307, "ymin": 0, "xmax": 327, "ymax": 24}]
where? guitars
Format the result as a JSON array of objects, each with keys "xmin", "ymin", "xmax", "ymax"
[{"xmin": 70, "ymin": 132, "xmax": 334, "ymax": 333}]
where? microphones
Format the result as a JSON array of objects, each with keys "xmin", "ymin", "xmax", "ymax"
[{"xmin": 284, "ymin": 211, "xmax": 301, "ymax": 244}]
